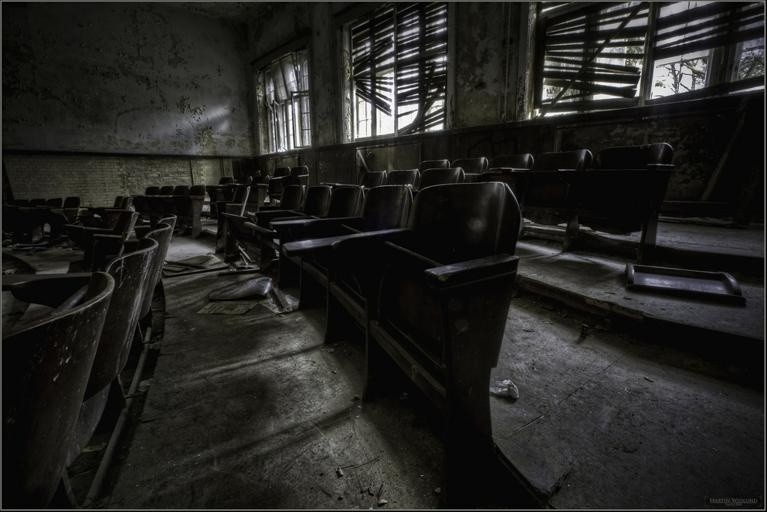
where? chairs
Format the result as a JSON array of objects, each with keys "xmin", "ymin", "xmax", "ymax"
[{"xmin": 1, "ymin": 141, "xmax": 679, "ymax": 512}]
